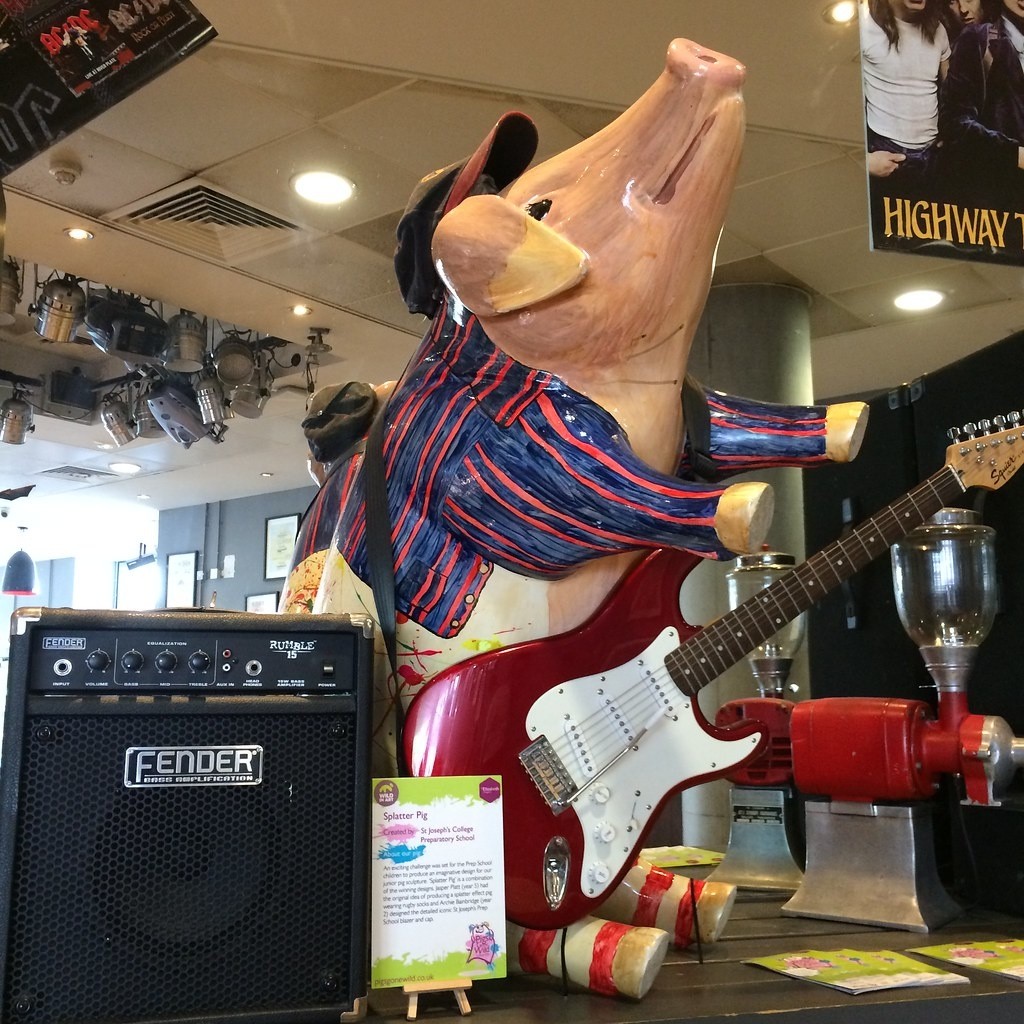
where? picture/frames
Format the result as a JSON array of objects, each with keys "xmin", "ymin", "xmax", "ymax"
[
  {"xmin": 263, "ymin": 512, "xmax": 303, "ymax": 582},
  {"xmin": 245, "ymin": 590, "xmax": 281, "ymax": 612},
  {"xmin": 164, "ymin": 550, "xmax": 201, "ymax": 607}
]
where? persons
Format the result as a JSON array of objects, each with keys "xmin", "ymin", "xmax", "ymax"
[{"xmin": 860, "ymin": 0, "xmax": 1024, "ymax": 196}]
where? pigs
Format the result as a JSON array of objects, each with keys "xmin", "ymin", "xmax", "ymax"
[{"xmin": 276, "ymin": 36, "xmax": 747, "ymax": 999}]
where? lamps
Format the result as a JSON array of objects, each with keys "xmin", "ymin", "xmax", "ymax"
[
  {"xmin": 3, "ymin": 548, "xmax": 38, "ymax": 610},
  {"xmin": 0, "ymin": 247, "xmax": 330, "ymax": 448},
  {"xmin": 125, "ymin": 543, "xmax": 155, "ymax": 572}
]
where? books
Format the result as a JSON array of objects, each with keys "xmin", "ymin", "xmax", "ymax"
[{"xmin": 741, "ymin": 936, "xmax": 1024, "ymax": 997}]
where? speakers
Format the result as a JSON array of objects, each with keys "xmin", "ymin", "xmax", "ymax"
[{"xmin": 0, "ymin": 605, "xmax": 376, "ymax": 1024}]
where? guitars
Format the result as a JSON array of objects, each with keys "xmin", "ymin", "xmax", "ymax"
[{"xmin": 399, "ymin": 410, "xmax": 1024, "ymax": 930}]
